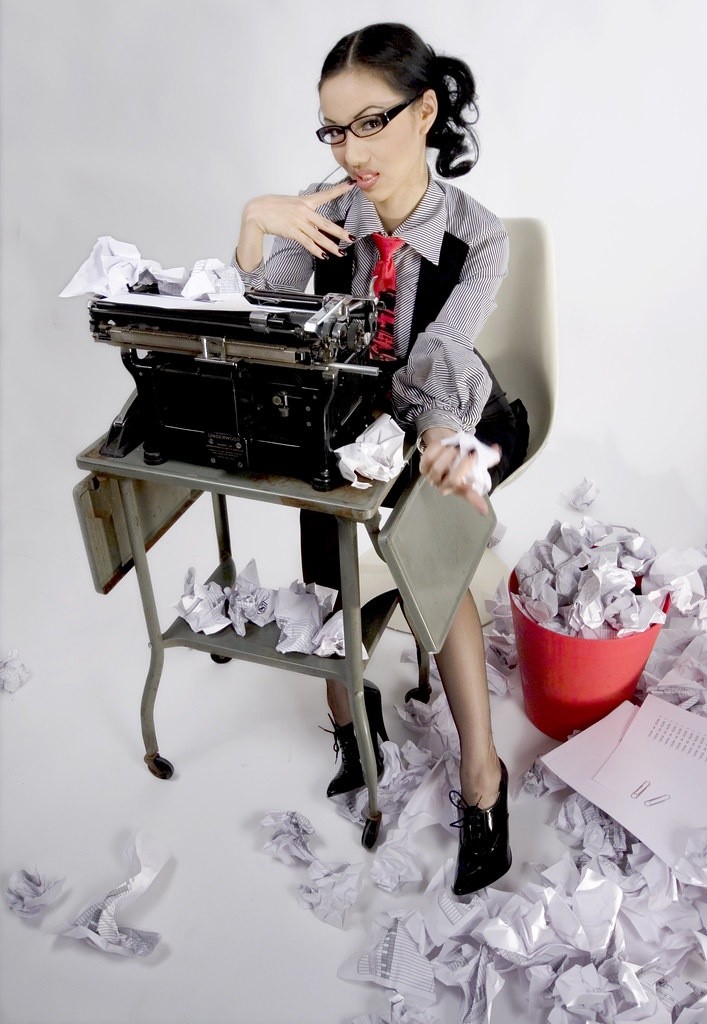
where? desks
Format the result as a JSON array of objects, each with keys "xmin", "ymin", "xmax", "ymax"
[{"xmin": 75, "ymin": 433, "xmax": 431, "ymax": 853}]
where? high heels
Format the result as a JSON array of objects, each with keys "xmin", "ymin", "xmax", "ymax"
[
  {"xmin": 451, "ymin": 757, "xmax": 513, "ymax": 895},
  {"xmin": 326, "ymin": 684, "xmax": 391, "ymax": 797}
]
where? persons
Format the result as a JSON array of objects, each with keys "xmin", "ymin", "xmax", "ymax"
[{"xmin": 231, "ymin": 22, "xmax": 528, "ymax": 898}]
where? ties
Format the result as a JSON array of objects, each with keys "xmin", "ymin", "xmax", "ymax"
[{"xmin": 368, "ymin": 233, "xmax": 409, "ymax": 363}]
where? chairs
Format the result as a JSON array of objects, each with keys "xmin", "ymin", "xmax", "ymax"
[{"xmin": 299, "ymin": 213, "xmax": 554, "ymax": 706}]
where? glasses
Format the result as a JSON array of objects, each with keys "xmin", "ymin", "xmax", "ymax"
[{"xmin": 316, "ymin": 88, "xmax": 429, "ymax": 145}]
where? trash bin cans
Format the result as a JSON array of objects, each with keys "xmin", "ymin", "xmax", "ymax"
[{"xmin": 508, "ymin": 545, "xmax": 671, "ymax": 743}]
